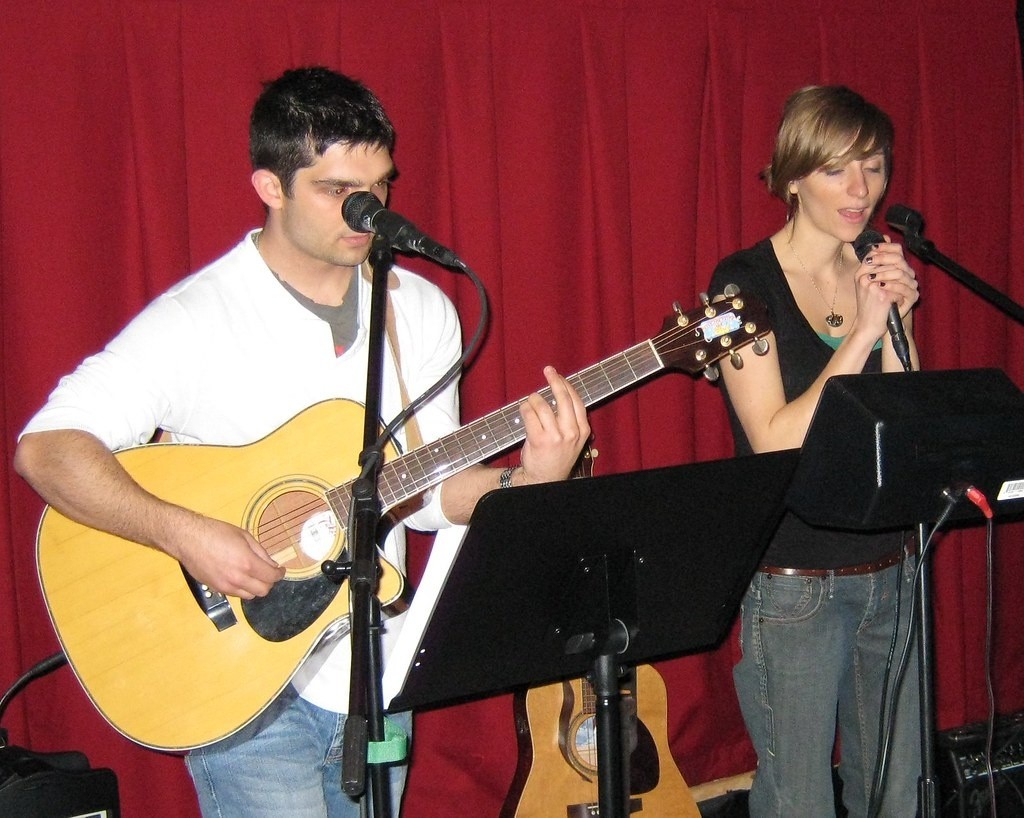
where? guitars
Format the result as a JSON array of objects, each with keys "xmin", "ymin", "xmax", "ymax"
[
  {"xmin": 497, "ymin": 427, "xmax": 702, "ymax": 818},
  {"xmin": 31, "ymin": 285, "xmax": 776, "ymax": 756}
]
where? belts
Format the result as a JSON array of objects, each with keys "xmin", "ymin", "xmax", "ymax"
[{"xmin": 756, "ymin": 537, "xmax": 916, "ymax": 576}]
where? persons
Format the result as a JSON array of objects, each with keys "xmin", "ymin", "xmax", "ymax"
[
  {"xmin": 14, "ymin": 64, "xmax": 591, "ymax": 818},
  {"xmin": 705, "ymin": 83, "xmax": 925, "ymax": 818}
]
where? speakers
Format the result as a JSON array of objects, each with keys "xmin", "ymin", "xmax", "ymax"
[{"xmin": 930, "ymin": 712, "xmax": 1024, "ymax": 818}]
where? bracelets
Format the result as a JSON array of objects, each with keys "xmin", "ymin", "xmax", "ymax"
[{"xmin": 501, "ymin": 464, "xmax": 518, "ymax": 488}]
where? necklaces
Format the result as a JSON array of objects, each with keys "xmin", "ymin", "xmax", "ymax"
[{"xmin": 786, "ymin": 218, "xmax": 844, "ymax": 330}]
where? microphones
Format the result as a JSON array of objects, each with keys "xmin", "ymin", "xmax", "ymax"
[
  {"xmin": 341, "ymin": 191, "xmax": 461, "ymax": 268},
  {"xmin": 854, "ymin": 230, "xmax": 912, "ymax": 374}
]
